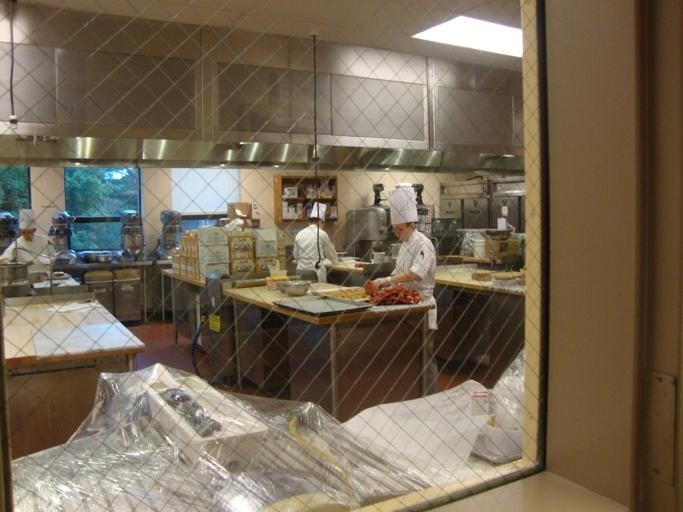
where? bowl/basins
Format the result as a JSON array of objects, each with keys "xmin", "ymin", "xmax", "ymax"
[
  {"xmin": 485, "ymin": 229, "xmax": 513, "ymax": 241},
  {"xmin": 282, "ymin": 280, "xmax": 311, "ymax": 296},
  {"xmin": 96, "ymin": 255, "xmax": 112, "ymax": 263}
]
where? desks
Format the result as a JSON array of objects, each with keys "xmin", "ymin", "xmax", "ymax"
[{"xmin": 0, "ymin": 291, "xmax": 146, "ymax": 457}]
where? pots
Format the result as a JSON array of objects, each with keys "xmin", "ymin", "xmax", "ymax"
[{"xmin": 0, "ymin": 260, "xmax": 34, "ymax": 283}]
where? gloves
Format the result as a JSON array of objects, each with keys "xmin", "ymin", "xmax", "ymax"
[{"xmin": 372, "ymin": 276, "xmax": 393, "ymax": 290}]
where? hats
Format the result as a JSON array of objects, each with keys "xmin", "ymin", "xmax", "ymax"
[
  {"xmin": 390, "ymin": 188, "xmax": 419, "ymax": 225},
  {"xmin": 310, "ymin": 202, "xmax": 327, "ymax": 222},
  {"xmin": 19, "ymin": 209, "xmax": 36, "ymax": 230}
]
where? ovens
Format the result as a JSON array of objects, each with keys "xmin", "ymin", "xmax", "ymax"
[{"xmin": 439, "ymin": 177, "xmax": 524, "ymax": 232}]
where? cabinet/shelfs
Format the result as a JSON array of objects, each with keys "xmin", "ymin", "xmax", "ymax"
[
  {"xmin": 493, "ymin": 195, "xmax": 525, "ymax": 233},
  {"xmin": 439, "ymin": 197, "xmax": 490, "ymax": 228},
  {"xmin": 84, "ymin": 278, "xmax": 141, "ymax": 322},
  {"xmin": 273, "ymin": 175, "xmax": 340, "ymax": 224}
]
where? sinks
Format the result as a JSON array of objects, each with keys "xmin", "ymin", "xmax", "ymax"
[{"xmin": 69, "ymin": 252, "xmax": 116, "ymax": 264}]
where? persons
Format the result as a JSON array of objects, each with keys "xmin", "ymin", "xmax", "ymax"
[
  {"xmin": 367, "ymin": 221, "xmax": 440, "ymax": 396},
  {"xmin": 292, "ymin": 218, "xmax": 340, "ymax": 285},
  {"xmin": 0, "ymin": 228, "xmax": 56, "ymax": 277}
]
